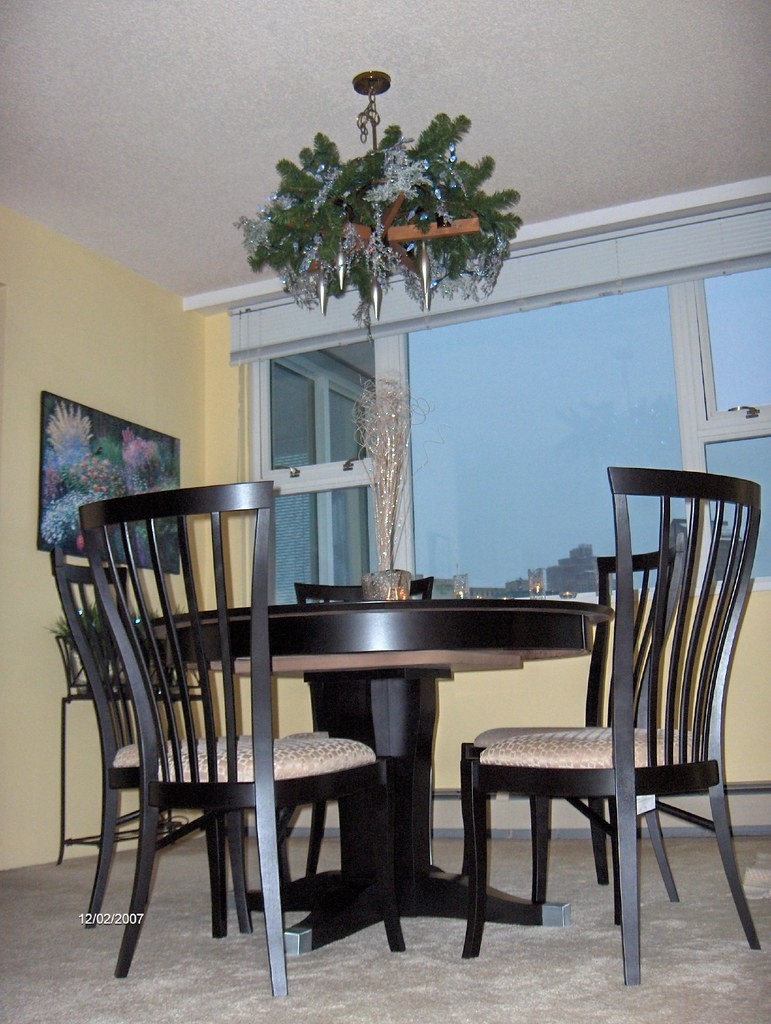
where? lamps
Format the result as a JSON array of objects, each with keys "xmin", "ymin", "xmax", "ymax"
[{"xmin": 231, "ymin": 70, "xmax": 524, "ymax": 344}]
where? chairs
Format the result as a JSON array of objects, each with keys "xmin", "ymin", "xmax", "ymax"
[
  {"xmin": 50, "ymin": 480, "xmax": 434, "ymax": 995},
  {"xmin": 460, "ymin": 464, "xmax": 761, "ymax": 986}
]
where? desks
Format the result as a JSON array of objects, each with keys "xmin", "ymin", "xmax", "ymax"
[{"xmin": 142, "ymin": 598, "xmax": 618, "ymax": 957}]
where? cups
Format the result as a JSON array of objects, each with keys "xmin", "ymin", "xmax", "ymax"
[
  {"xmin": 453, "ymin": 574, "xmax": 471, "ymax": 599},
  {"xmin": 528, "ymin": 568, "xmax": 547, "ymax": 600}
]
